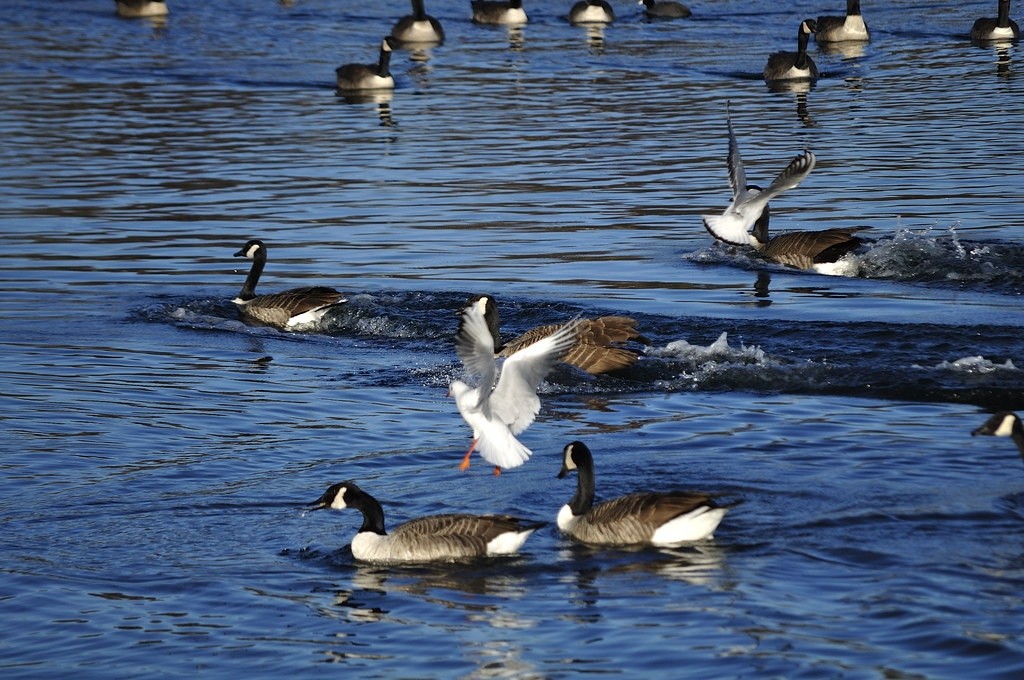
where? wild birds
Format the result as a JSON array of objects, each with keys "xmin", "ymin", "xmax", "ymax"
[
  {"xmin": 233, "ymin": 240, "xmax": 348, "ymax": 330},
  {"xmin": 638, "ymin": 0, "xmax": 693, "ymax": 17},
  {"xmin": 335, "ymin": 37, "xmax": 406, "ymax": 89},
  {"xmin": 569, "ymin": 0, "xmax": 616, "ymax": 22},
  {"xmin": 113, "ymin": 0, "xmax": 169, "ymax": 17},
  {"xmin": 471, "ymin": 0, "xmax": 528, "ymax": 24},
  {"xmin": 728, "ymin": 185, "xmax": 874, "ymax": 270},
  {"xmin": 970, "ymin": 0, "xmax": 1019, "ymax": 40},
  {"xmin": 307, "ymin": 482, "xmax": 548, "ymax": 562},
  {"xmin": 445, "ymin": 298, "xmax": 589, "ymax": 477},
  {"xmin": 557, "ymin": 442, "xmax": 743, "ymax": 547},
  {"xmin": 703, "ymin": 99, "xmax": 815, "ymax": 255},
  {"xmin": 815, "ymin": 0, "xmax": 871, "ymax": 42},
  {"xmin": 392, "ymin": 0, "xmax": 446, "ymax": 41},
  {"xmin": 763, "ymin": 18, "xmax": 822, "ymax": 80},
  {"xmin": 496, "ymin": 313, "xmax": 652, "ymax": 379},
  {"xmin": 971, "ymin": 411, "xmax": 1024, "ymax": 459}
]
